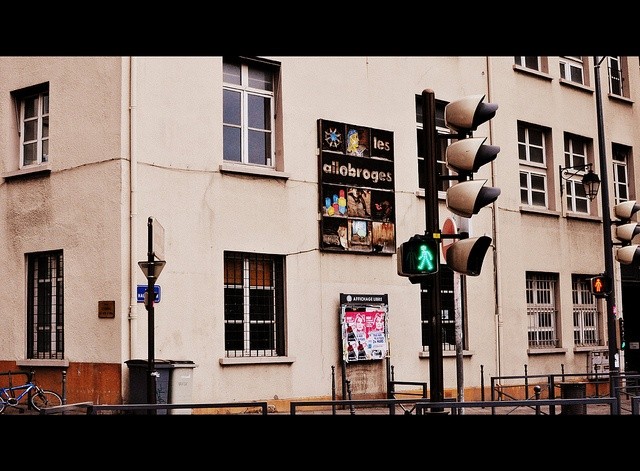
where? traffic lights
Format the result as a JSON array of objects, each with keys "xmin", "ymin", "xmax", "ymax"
[
  {"xmin": 591, "ymin": 275, "xmax": 611, "ymax": 295},
  {"xmin": 447, "ymin": 236, "xmax": 492, "ymax": 276},
  {"xmin": 615, "ymin": 318, "xmax": 626, "ymax": 350},
  {"xmin": 444, "ymin": 94, "xmax": 501, "ymax": 218},
  {"xmin": 614, "ymin": 200, "xmax": 640, "ymax": 264},
  {"xmin": 397, "ymin": 235, "xmax": 439, "ymax": 275}
]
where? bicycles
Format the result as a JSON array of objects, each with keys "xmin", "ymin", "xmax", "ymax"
[{"xmin": 0, "ymin": 366, "xmax": 62, "ymax": 414}]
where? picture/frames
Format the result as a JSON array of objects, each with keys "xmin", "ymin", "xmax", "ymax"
[{"xmin": 318, "ymin": 118, "xmax": 396, "ymax": 257}]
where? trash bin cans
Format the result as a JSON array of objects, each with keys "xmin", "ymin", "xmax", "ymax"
[
  {"xmin": 124, "ymin": 359, "xmax": 174, "ymax": 415},
  {"xmin": 165, "ymin": 360, "xmax": 197, "ymax": 415},
  {"xmin": 560, "ymin": 383, "xmax": 587, "ymax": 415}
]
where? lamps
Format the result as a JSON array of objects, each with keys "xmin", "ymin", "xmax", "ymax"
[{"xmin": 559, "ymin": 162, "xmax": 599, "ymax": 202}]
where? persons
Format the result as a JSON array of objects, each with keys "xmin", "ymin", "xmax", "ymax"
[
  {"xmin": 369, "ymin": 313, "xmax": 385, "ymax": 343},
  {"xmin": 354, "ymin": 313, "xmax": 366, "ymax": 339}
]
